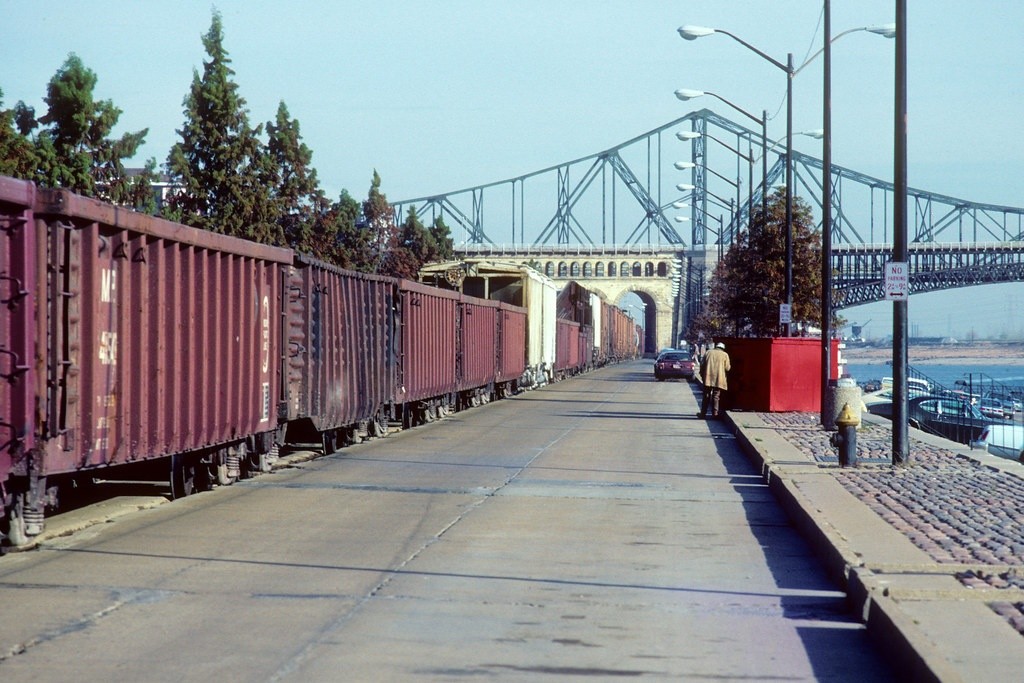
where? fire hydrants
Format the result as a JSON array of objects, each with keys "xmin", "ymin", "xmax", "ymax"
[{"xmin": 828, "ymin": 402, "xmax": 860, "ymax": 468}]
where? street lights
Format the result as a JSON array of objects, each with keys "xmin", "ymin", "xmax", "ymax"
[
  {"xmin": 678, "ymin": 22, "xmax": 895, "ymax": 339},
  {"xmin": 672, "ymin": 88, "xmax": 824, "ymax": 309}
]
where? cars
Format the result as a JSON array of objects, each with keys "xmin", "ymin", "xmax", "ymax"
[
  {"xmin": 654, "ymin": 348, "xmax": 696, "ymax": 384},
  {"xmin": 861, "ymin": 375, "xmax": 1024, "ymax": 467}
]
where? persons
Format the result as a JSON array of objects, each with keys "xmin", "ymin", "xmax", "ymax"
[
  {"xmin": 696, "ymin": 342, "xmax": 731, "ymax": 420},
  {"xmin": 693, "ymin": 342, "xmax": 700, "ymax": 365},
  {"xmin": 699, "ymin": 344, "xmax": 706, "ymax": 365}
]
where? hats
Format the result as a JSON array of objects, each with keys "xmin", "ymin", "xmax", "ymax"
[{"xmin": 716, "ymin": 342, "xmax": 726, "ymax": 349}]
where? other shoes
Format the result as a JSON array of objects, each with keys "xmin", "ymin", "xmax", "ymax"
[
  {"xmin": 697, "ymin": 413, "xmax": 705, "ymax": 419},
  {"xmin": 712, "ymin": 414, "xmax": 721, "ymax": 420}
]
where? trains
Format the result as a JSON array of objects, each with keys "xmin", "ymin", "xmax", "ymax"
[{"xmin": 0, "ymin": 167, "xmax": 644, "ymax": 557}]
